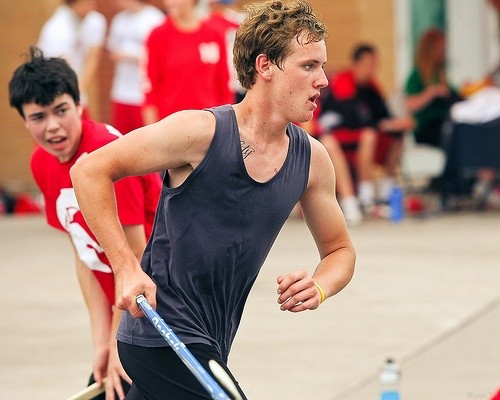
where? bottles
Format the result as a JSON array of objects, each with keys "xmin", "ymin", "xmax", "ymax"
[
  {"xmin": 389, "ymin": 187, "xmax": 404, "ymax": 221},
  {"xmin": 378, "ymin": 359, "xmax": 402, "ymax": 400}
]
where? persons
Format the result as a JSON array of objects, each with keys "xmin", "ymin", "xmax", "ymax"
[
  {"xmin": 36, "ymin": 0, "xmax": 500, "ymax": 225},
  {"xmin": 9, "ymin": 48, "xmax": 164, "ymax": 400},
  {"xmin": 69, "ymin": 0, "xmax": 356, "ymax": 400}
]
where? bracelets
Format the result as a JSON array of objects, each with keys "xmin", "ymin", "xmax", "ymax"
[{"xmin": 311, "ymin": 279, "xmax": 325, "ymax": 302}]
